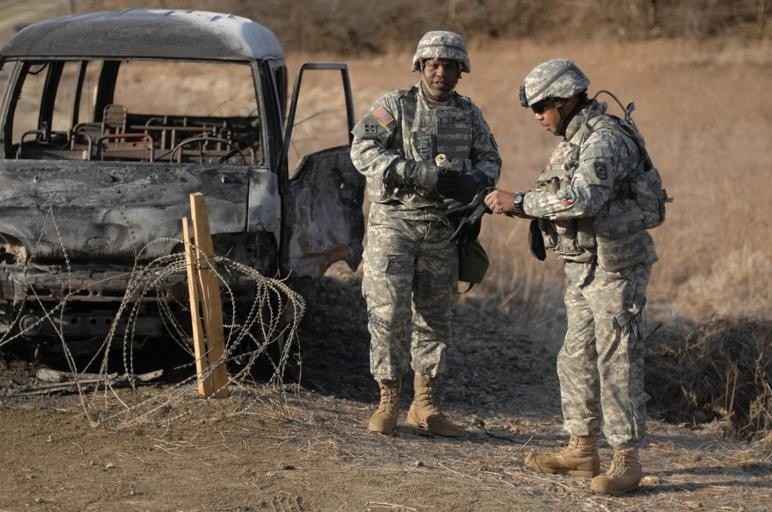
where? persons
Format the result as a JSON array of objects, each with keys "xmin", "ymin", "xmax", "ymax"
[
  {"xmin": 350, "ymin": 30, "xmax": 502, "ymax": 437},
  {"xmin": 484, "ymin": 59, "xmax": 665, "ymax": 497}
]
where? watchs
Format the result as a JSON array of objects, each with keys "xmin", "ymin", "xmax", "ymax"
[{"xmin": 513, "ymin": 192, "xmax": 526, "ymax": 216}]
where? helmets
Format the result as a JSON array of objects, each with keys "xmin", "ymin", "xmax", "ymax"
[
  {"xmin": 411, "ymin": 30, "xmax": 470, "ymax": 72},
  {"xmin": 519, "ymin": 58, "xmax": 590, "ymax": 107}
]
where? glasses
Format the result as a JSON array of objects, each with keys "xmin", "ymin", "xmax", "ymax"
[{"xmin": 531, "ymin": 102, "xmax": 544, "ymax": 113}]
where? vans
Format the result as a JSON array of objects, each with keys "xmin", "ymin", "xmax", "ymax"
[{"xmin": 0, "ymin": 10, "xmax": 365, "ymax": 372}]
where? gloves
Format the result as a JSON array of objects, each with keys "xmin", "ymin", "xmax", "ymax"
[{"xmin": 403, "ymin": 158, "xmax": 492, "ymax": 245}]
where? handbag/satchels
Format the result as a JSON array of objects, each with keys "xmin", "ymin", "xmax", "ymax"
[{"xmin": 458, "ymin": 238, "xmax": 490, "ymax": 284}]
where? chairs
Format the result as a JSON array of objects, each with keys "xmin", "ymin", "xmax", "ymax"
[{"xmin": 17, "ymin": 102, "xmax": 260, "ymax": 168}]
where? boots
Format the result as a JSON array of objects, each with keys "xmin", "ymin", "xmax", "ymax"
[
  {"xmin": 368, "ymin": 380, "xmax": 402, "ymax": 434},
  {"xmin": 524, "ymin": 434, "xmax": 600, "ymax": 478},
  {"xmin": 590, "ymin": 446, "xmax": 642, "ymax": 496},
  {"xmin": 407, "ymin": 373, "xmax": 458, "ymax": 438}
]
